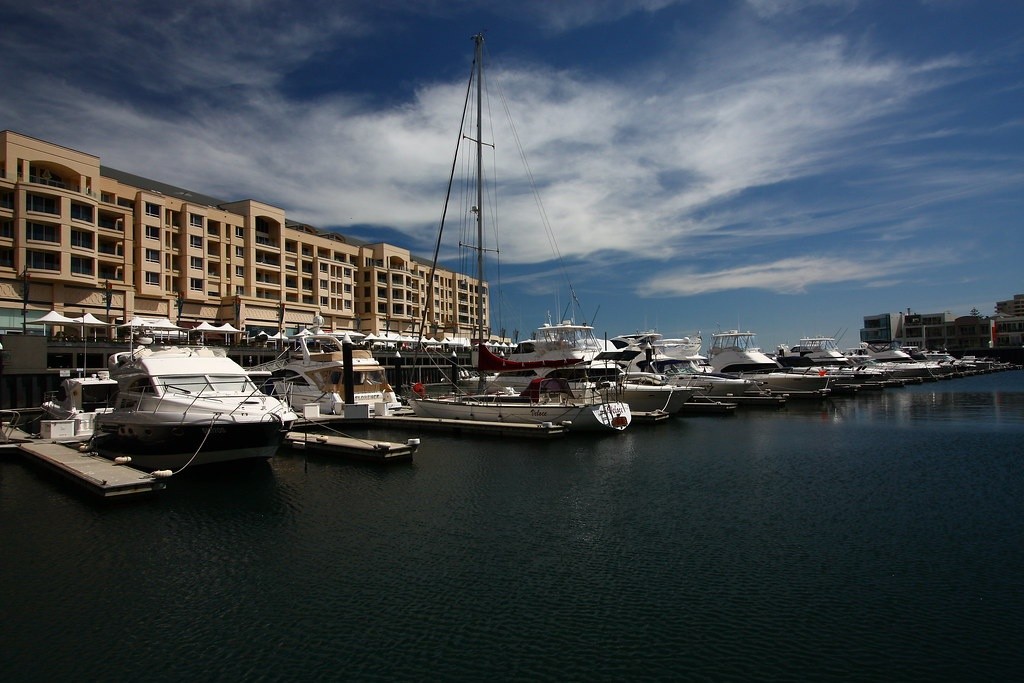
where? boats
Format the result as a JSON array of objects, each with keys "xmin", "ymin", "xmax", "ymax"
[{"xmin": 0, "ymin": 29, "xmax": 1024, "ymax": 481}]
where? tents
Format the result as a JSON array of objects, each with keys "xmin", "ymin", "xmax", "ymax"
[{"xmin": 17, "ymin": 308, "xmax": 519, "ymax": 354}]
